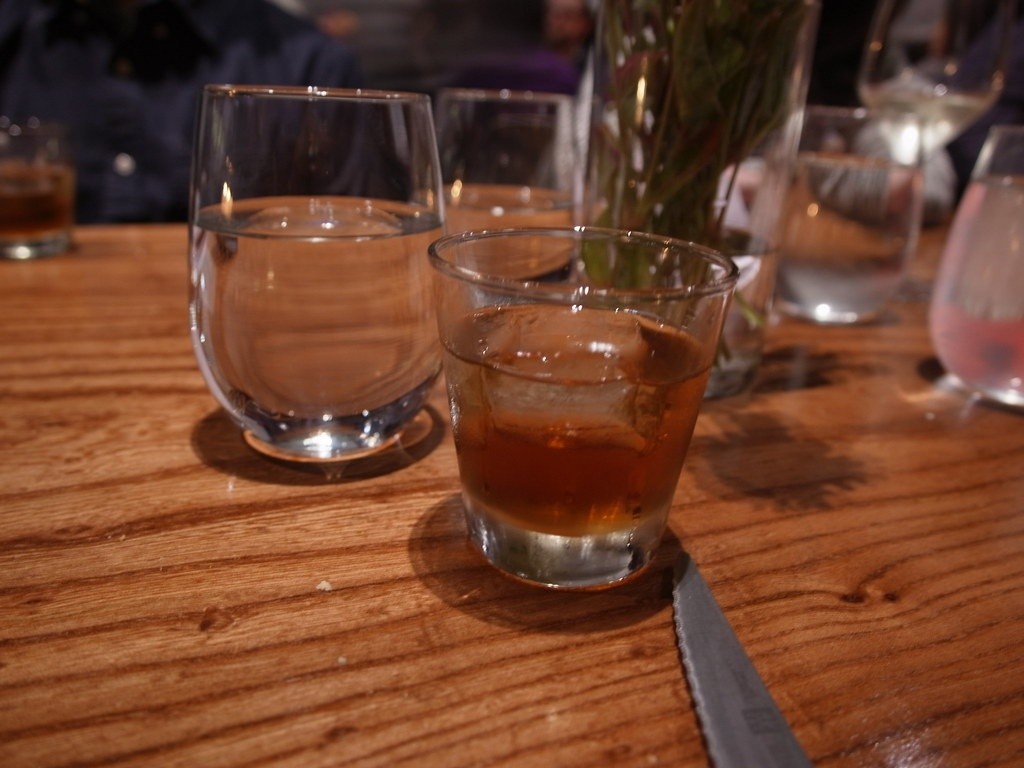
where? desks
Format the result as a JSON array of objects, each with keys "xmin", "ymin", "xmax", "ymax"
[{"xmin": 0, "ymin": 219, "xmax": 1024, "ymax": 768}]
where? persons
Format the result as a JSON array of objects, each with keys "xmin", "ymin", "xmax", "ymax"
[
  {"xmin": 0, "ymin": 0, "xmax": 414, "ymax": 223},
  {"xmin": 438, "ymin": 0, "xmax": 595, "ymax": 183}
]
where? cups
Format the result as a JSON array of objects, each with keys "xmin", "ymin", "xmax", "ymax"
[
  {"xmin": 427, "ymin": 89, "xmax": 577, "ymax": 287},
  {"xmin": 774, "ymin": 104, "xmax": 924, "ymax": 323},
  {"xmin": 426, "ymin": 223, "xmax": 739, "ymax": 591},
  {"xmin": 925, "ymin": 127, "xmax": 1023, "ymax": 407},
  {"xmin": 0, "ymin": 122, "xmax": 79, "ymax": 260},
  {"xmin": 186, "ymin": 81, "xmax": 452, "ymax": 464}
]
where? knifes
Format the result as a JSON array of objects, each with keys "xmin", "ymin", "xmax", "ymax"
[{"xmin": 664, "ymin": 549, "xmax": 816, "ymax": 768}]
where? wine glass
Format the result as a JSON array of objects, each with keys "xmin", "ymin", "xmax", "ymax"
[{"xmin": 857, "ymin": 1, "xmax": 1018, "ymax": 215}]
